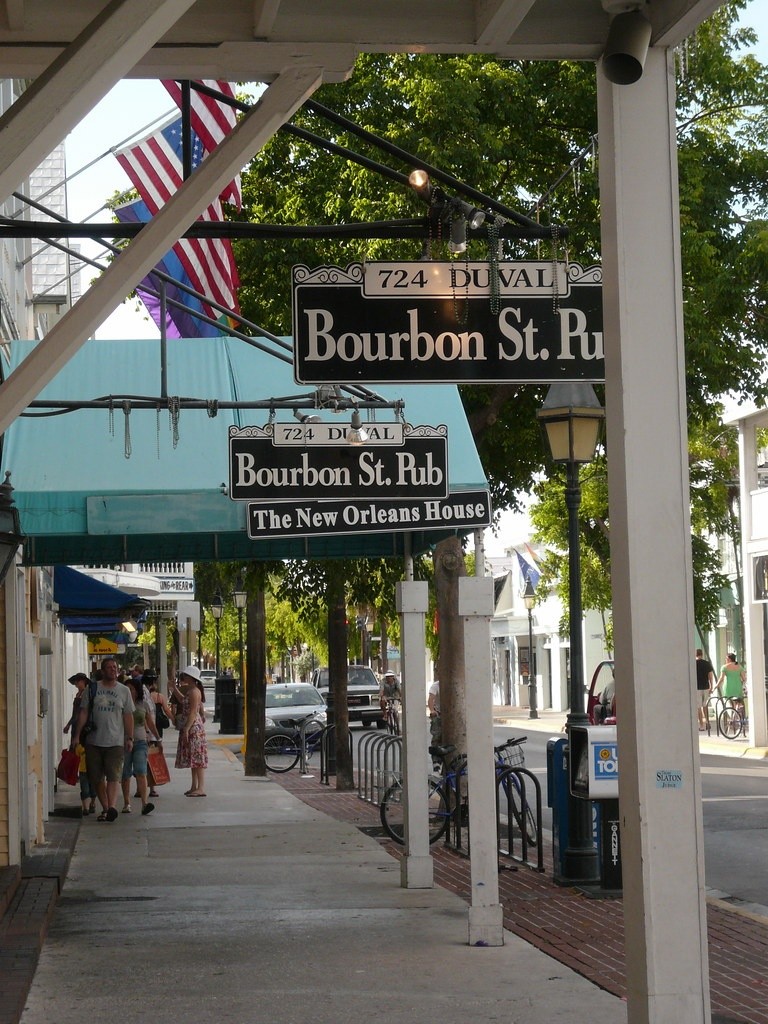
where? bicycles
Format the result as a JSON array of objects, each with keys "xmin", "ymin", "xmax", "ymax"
[
  {"xmin": 718, "ymin": 696, "xmax": 750, "ymax": 739},
  {"xmin": 379, "ymin": 735, "xmax": 538, "ymax": 860},
  {"xmin": 378, "ymin": 695, "xmax": 402, "ymax": 735},
  {"xmin": 263, "ymin": 712, "xmax": 328, "ymax": 773}
]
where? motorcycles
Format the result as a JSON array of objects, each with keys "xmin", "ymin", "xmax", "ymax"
[{"xmin": 586, "ymin": 659, "xmax": 617, "ymax": 726}]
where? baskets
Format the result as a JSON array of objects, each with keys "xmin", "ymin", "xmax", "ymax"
[{"xmin": 494, "ymin": 744, "xmax": 526, "ymax": 775}]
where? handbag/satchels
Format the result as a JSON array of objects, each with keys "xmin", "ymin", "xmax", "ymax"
[
  {"xmin": 147, "ymin": 744, "xmax": 170, "ymax": 786},
  {"xmin": 158, "ymin": 712, "xmax": 169, "ymax": 729},
  {"xmin": 58, "ymin": 748, "xmax": 79, "ymax": 785}
]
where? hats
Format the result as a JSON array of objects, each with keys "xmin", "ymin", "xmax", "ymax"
[
  {"xmin": 68, "ymin": 673, "xmax": 90, "ymax": 685},
  {"xmin": 385, "ymin": 670, "xmax": 395, "ymax": 676},
  {"xmin": 182, "ymin": 666, "xmax": 204, "ymax": 683},
  {"xmin": 141, "ymin": 669, "xmax": 159, "ymax": 678}
]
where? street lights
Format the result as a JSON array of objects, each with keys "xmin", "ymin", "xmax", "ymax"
[
  {"xmin": 364, "ymin": 614, "xmax": 374, "ymax": 669},
  {"xmin": 536, "ymin": 382, "xmax": 606, "ymax": 887},
  {"xmin": 197, "ymin": 607, "xmax": 205, "ymax": 669},
  {"xmin": 230, "ymin": 578, "xmax": 247, "ymax": 734},
  {"xmin": 211, "ymin": 589, "xmax": 225, "ymax": 678},
  {"xmin": 520, "ymin": 575, "xmax": 539, "ymax": 719}
]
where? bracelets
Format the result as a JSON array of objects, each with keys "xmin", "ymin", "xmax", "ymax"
[{"xmin": 126, "ymin": 737, "xmax": 134, "ymax": 743}]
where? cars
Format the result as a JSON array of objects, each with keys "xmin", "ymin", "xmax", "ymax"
[{"xmin": 265, "ymin": 683, "xmax": 328, "ymax": 749}]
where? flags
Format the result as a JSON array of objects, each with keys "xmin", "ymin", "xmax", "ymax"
[
  {"xmin": 111, "ymin": 111, "xmax": 240, "ymax": 322},
  {"xmin": 111, "ymin": 197, "xmax": 239, "ymax": 340},
  {"xmin": 159, "ymin": 77, "xmax": 243, "ymax": 214}
]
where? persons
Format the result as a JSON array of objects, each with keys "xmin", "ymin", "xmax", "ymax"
[
  {"xmin": 593, "ymin": 669, "xmax": 616, "ymax": 726},
  {"xmin": 71, "ymin": 658, "xmax": 134, "ymax": 822},
  {"xmin": 63, "ymin": 665, "xmax": 191, "ymax": 816},
  {"xmin": 711, "ymin": 653, "xmax": 747, "ymax": 738},
  {"xmin": 167, "ymin": 666, "xmax": 207, "ymax": 797},
  {"xmin": 696, "ymin": 648, "xmax": 714, "ymax": 732},
  {"xmin": 428, "ymin": 681, "xmax": 442, "ymax": 772},
  {"xmin": 378, "ymin": 669, "xmax": 402, "ymax": 733}
]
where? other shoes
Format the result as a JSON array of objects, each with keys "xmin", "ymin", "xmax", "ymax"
[
  {"xmin": 134, "ymin": 792, "xmax": 141, "ymax": 797},
  {"xmin": 105, "ymin": 807, "xmax": 118, "ymax": 822},
  {"xmin": 83, "ymin": 802, "xmax": 95, "ymax": 816},
  {"xmin": 97, "ymin": 810, "xmax": 108, "ymax": 821},
  {"xmin": 186, "ymin": 793, "xmax": 206, "ymax": 796},
  {"xmin": 184, "ymin": 790, "xmax": 192, "ymax": 795},
  {"xmin": 149, "ymin": 791, "xmax": 159, "ymax": 797},
  {"xmin": 142, "ymin": 803, "xmax": 154, "ymax": 814},
  {"xmin": 122, "ymin": 805, "xmax": 132, "ymax": 812},
  {"xmin": 699, "ymin": 723, "xmax": 711, "ymax": 732}
]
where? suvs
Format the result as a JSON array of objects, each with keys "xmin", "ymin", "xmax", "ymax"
[
  {"xmin": 200, "ymin": 671, "xmax": 217, "ymax": 687},
  {"xmin": 310, "ymin": 665, "xmax": 388, "ymax": 730}
]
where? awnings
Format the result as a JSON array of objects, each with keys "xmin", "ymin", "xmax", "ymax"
[{"xmin": 52, "ymin": 565, "xmax": 148, "ymax": 645}]
